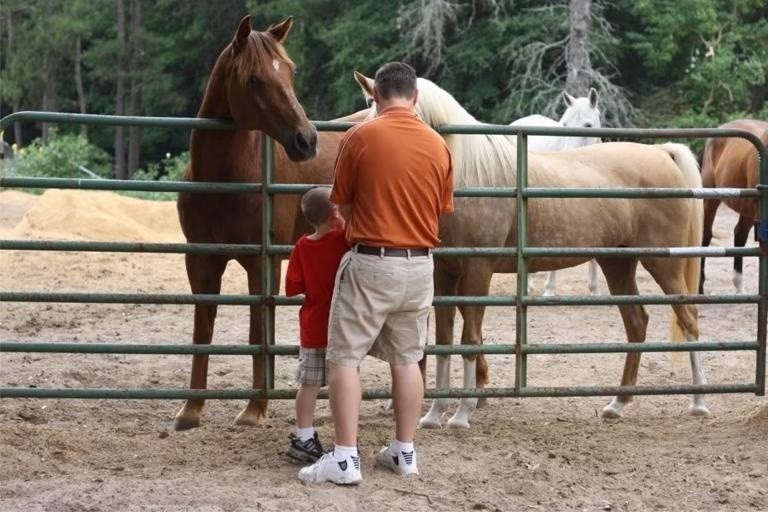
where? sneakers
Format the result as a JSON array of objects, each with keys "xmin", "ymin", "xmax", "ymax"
[
  {"xmin": 298, "ymin": 452, "xmax": 362, "ymax": 486},
  {"xmin": 378, "ymin": 444, "xmax": 419, "ymax": 476},
  {"xmin": 287, "ymin": 431, "xmax": 324, "ymax": 462}
]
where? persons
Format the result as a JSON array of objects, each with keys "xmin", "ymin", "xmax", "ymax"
[
  {"xmin": 298, "ymin": 62, "xmax": 455, "ymax": 486},
  {"xmin": 285, "ymin": 188, "xmax": 362, "ymax": 466}
]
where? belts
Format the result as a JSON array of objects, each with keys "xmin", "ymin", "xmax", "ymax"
[{"xmin": 353, "ymin": 244, "xmax": 430, "ymax": 258}]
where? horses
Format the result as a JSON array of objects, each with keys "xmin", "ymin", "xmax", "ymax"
[
  {"xmin": 172, "ymin": 14, "xmax": 429, "ymax": 430},
  {"xmin": 353, "ymin": 70, "xmax": 710, "ymax": 430},
  {"xmin": 697, "ymin": 119, "xmax": 768, "ymax": 318},
  {"xmin": 504, "ymin": 87, "xmax": 603, "ymax": 297}
]
